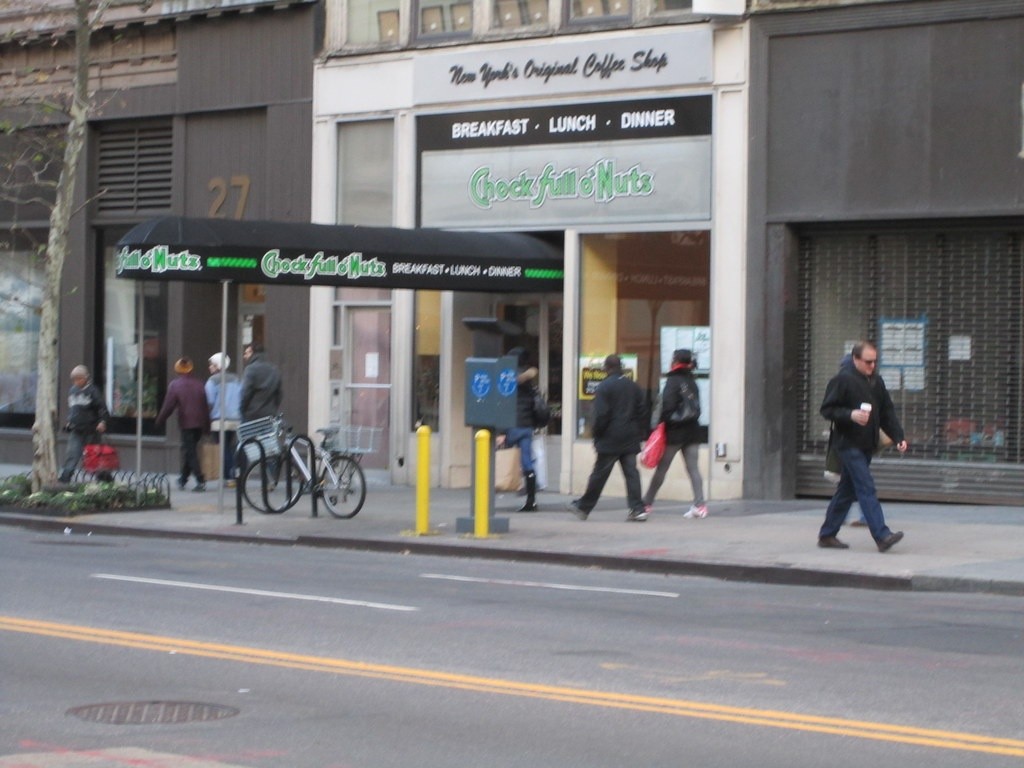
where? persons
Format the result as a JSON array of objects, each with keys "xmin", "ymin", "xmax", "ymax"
[
  {"xmin": 493, "ymin": 346, "xmax": 539, "ymax": 513},
  {"xmin": 154, "ymin": 357, "xmax": 209, "ymax": 492},
  {"xmin": 59, "ymin": 365, "xmax": 113, "ymax": 483},
  {"xmin": 240, "ymin": 342, "xmax": 283, "ymax": 482},
  {"xmin": 642, "ymin": 349, "xmax": 707, "ymax": 519},
  {"xmin": 569, "ymin": 355, "xmax": 646, "ymax": 521},
  {"xmin": 204, "ymin": 352, "xmax": 243, "ymax": 488},
  {"xmin": 819, "ymin": 340, "xmax": 907, "ymax": 552}
]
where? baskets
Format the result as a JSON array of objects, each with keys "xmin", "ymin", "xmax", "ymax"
[{"xmin": 236, "ymin": 415, "xmax": 281, "ymax": 461}]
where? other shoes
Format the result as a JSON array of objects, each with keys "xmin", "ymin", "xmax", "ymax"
[
  {"xmin": 176, "ymin": 477, "xmax": 185, "ymax": 488},
  {"xmin": 566, "ymin": 500, "xmax": 589, "ymax": 521},
  {"xmin": 193, "ymin": 481, "xmax": 207, "ymax": 492},
  {"xmin": 626, "ymin": 505, "xmax": 649, "ymax": 523},
  {"xmin": 683, "ymin": 506, "xmax": 707, "ymax": 519},
  {"xmin": 877, "ymin": 530, "xmax": 903, "ymax": 551},
  {"xmin": 817, "ymin": 535, "xmax": 849, "ymax": 550}
]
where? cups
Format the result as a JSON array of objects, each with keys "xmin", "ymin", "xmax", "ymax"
[{"xmin": 860, "ymin": 402, "xmax": 872, "ymax": 424}]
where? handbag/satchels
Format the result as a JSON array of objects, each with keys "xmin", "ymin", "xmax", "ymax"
[
  {"xmin": 81, "ymin": 435, "xmax": 121, "ymax": 475},
  {"xmin": 531, "ymin": 392, "xmax": 550, "ymax": 426},
  {"xmin": 640, "ymin": 423, "xmax": 668, "ymax": 468},
  {"xmin": 825, "ymin": 430, "xmax": 842, "ymax": 474},
  {"xmin": 494, "ymin": 443, "xmax": 523, "ymax": 490},
  {"xmin": 667, "ymin": 374, "xmax": 700, "ymax": 428}
]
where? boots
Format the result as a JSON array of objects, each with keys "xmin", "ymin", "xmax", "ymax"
[{"xmin": 518, "ymin": 470, "xmax": 539, "ymax": 513}]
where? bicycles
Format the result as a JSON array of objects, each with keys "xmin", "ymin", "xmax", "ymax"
[{"xmin": 237, "ymin": 410, "xmax": 368, "ymax": 521}]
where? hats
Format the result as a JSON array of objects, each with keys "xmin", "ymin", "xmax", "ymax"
[
  {"xmin": 209, "ymin": 352, "xmax": 231, "ymax": 371},
  {"xmin": 175, "ymin": 357, "xmax": 194, "ymax": 374}
]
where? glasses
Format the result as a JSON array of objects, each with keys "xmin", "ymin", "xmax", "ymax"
[{"xmin": 854, "ymin": 354, "xmax": 879, "ymax": 366}]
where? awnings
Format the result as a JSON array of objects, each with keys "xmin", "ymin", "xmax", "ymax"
[{"xmin": 116, "ymin": 215, "xmax": 563, "ymax": 515}]
